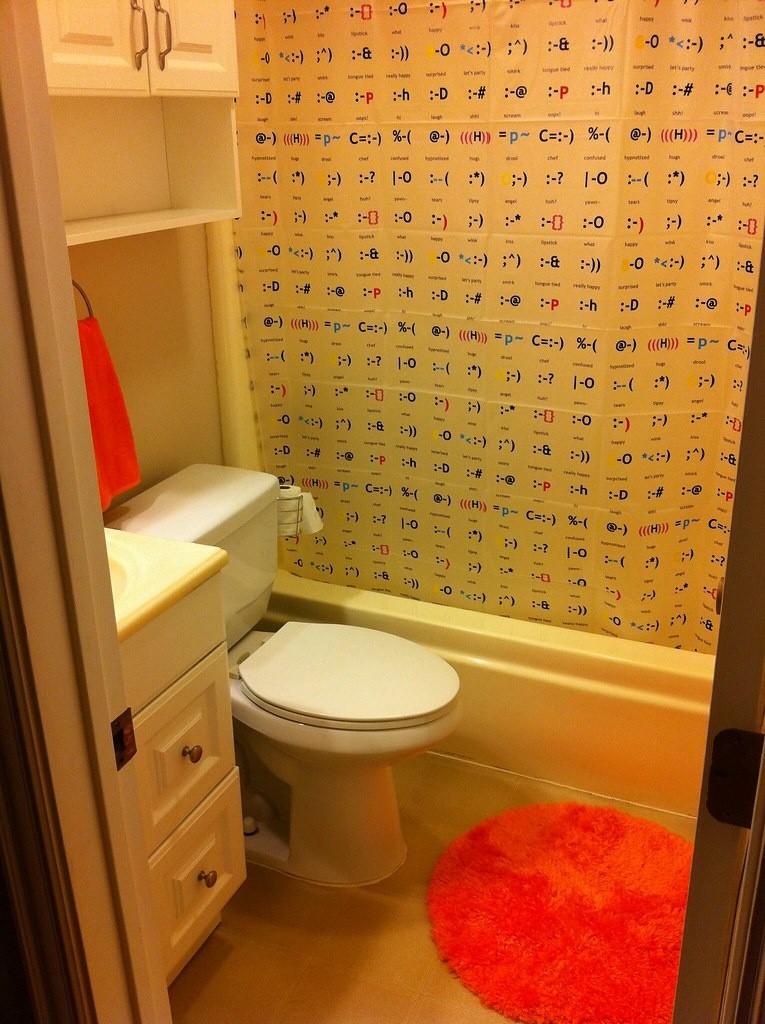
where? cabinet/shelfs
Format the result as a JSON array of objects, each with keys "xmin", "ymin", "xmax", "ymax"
[
  {"xmin": 38, "ymin": 0, "xmax": 244, "ymax": 246},
  {"xmin": 102, "ymin": 527, "xmax": 248, "ymax": 990}
]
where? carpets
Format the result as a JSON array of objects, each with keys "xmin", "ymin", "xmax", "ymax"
[{"xmin": 429, "ymin": 805, "xmax": 694, "ymax": 1024}]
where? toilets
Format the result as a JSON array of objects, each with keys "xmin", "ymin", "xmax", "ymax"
[{"xmin": 103, "ymin": 458, "xmax": 465, "ymax": 890}]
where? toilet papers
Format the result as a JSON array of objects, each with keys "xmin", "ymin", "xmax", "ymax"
[{"xmin": 278, "ymin": 484, "xmax": 324, "ymax": 537}]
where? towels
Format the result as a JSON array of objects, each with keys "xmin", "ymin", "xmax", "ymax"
[{"xmin": 74, "ymin": 313, "xmax": 143, "ymax": 515}]
